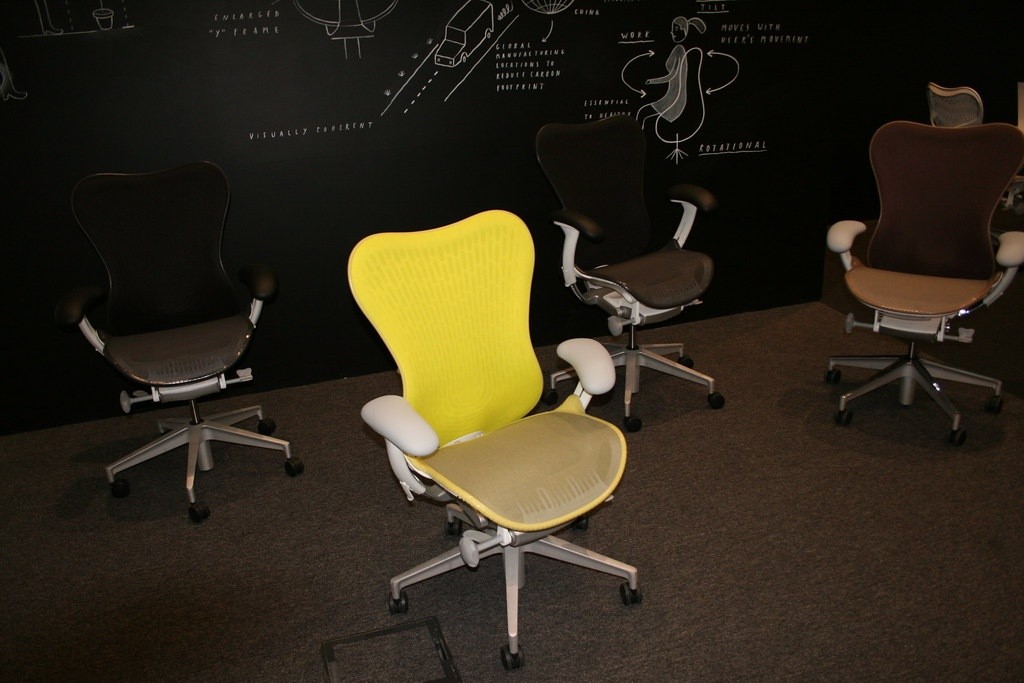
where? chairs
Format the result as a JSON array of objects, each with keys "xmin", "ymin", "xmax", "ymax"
[
  {"xmin": 348, "ymin": 209, "xmax": 643, "ymax": 670},
  {"xmin": 925, "ymin": 82, "xmax": 1024, "ymax": 212},
  {"xmin": 826, "ymin": 121, "xmax": 1024, "ymax": 445},
  {"xmin": 60, "ymin": 160, "xmax": 303, "ymax": 522},
  {"xmin": 536, "ymin": 114, "xmax": 725, "ymax": 433}
]
